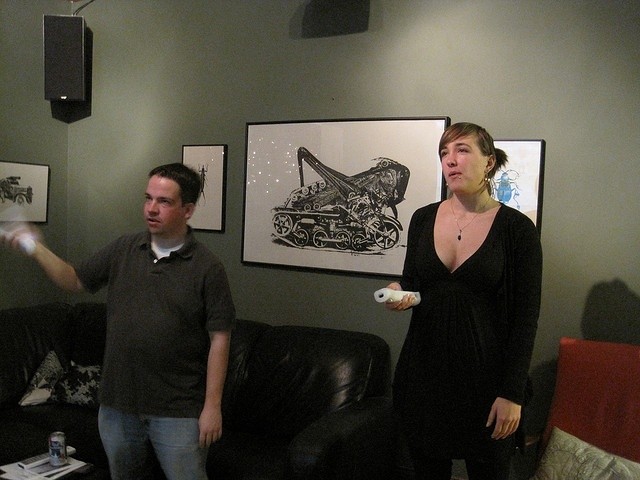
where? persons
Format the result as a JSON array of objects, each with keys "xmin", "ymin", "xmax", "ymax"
[
  {"xmin": 3, "ymin": 160, "xmax": 235, "ymax": 478},
  {"xmin": 382, "ymin": 121, "xmax": 543, "ymax": 477}
]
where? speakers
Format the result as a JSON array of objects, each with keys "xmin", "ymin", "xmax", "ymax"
[{"xmin": 41, "ymin": 14, "xmax": 86, "ymax": 101}]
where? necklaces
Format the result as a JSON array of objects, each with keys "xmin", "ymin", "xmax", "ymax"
[{"xmin": 449, "ymin": 197, "xmax": 491, "ymax": 242}]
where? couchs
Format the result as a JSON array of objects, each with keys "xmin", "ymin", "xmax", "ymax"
[
  {"xmin": 4, "ymin": 299, "xmax": 392, "ymax": 479},
  {"xmin": 544, "ymin": 337, "xmax": 640, "ymax": 465}
]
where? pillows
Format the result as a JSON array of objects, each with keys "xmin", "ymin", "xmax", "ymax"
[
  {"xmin": 18, "ymin": 348, "xmax": 101, "ymax": 409},
  {"xmin": 537, "ymin": 427, "xmax": 638, "ymax": 479}
]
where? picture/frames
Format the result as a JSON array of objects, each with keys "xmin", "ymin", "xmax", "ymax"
[
  {"xmin": 178, "ymin": 143, "xmax": 228, "ymax": 234},
  {"xmin": 0, "ymin": 159, "xmax": 53, "ymax": 226},
  {"xmin": 238, "ymin": 115, "xmax": 452, "ymax": 281},
  {"xmin": 491, "ymin": 138, "xmax": 548, "ymax": 235}
]
